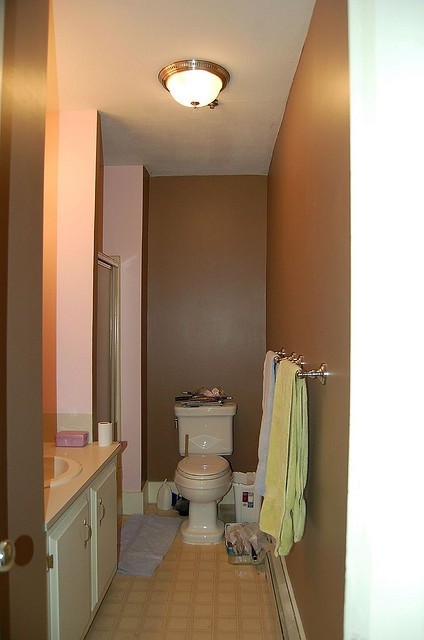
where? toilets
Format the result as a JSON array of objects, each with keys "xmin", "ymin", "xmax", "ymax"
[{"xmin": 175, "ymin": 400, "xmax": 237, "ymax": 546}]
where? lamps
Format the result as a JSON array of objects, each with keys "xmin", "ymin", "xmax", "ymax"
[{"xmin": 157, "ymin": 61, "xmax": 231, "ymax": 110}]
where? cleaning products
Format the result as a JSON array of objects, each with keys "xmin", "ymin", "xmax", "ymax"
[{"xmin": 156, "ymin": 479, "xmax": 173, "ymax": 510}]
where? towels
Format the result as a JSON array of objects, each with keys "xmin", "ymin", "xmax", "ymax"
[
  {"xmin": 259, "ymin": 359, "xmax": 309, "ymax": 557},
  {"xmin": 253, "ymin": 350, "xmax": 280, "ymax": 497}
]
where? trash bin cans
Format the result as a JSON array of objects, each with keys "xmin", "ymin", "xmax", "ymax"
[{"xmin": 232, "ymin": 471, "xmax": 262, "ymax": 524}]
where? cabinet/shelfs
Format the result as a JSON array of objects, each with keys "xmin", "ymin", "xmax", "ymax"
[{"xmin": 47, "ymin": 462, "xmax": 118, "ymax": 640}]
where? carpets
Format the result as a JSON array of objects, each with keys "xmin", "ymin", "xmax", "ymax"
[{"xmin": 116, "ymin": 512, "xmax": 183, "ymax": 577}]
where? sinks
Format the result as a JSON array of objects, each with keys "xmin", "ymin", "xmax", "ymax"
[{"xmin": 42, "ymin": 453, "xmax": 81, "ymax": 490}]
